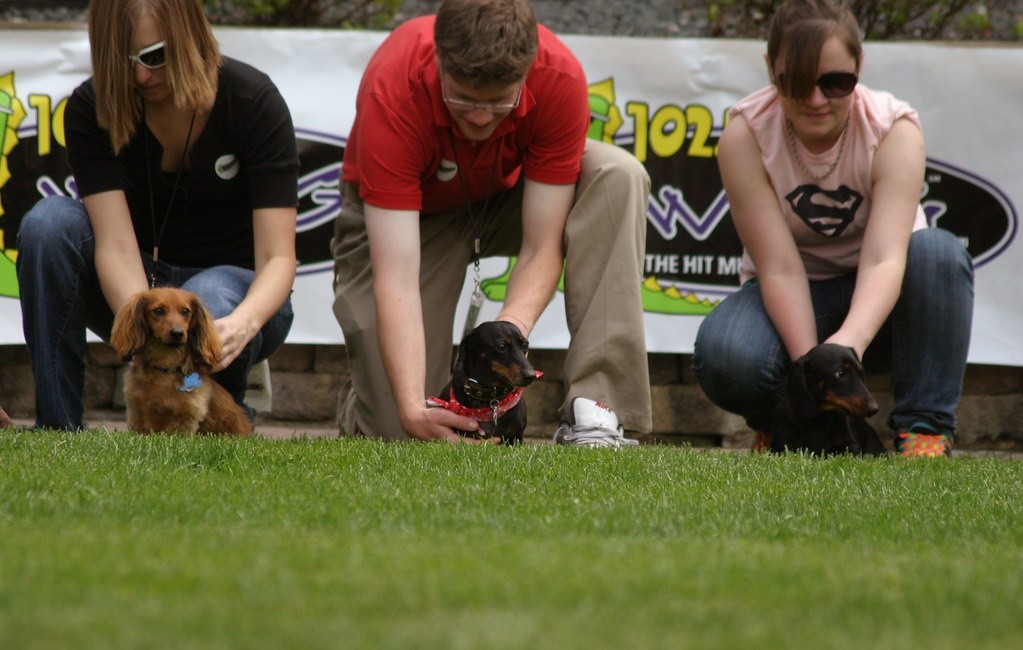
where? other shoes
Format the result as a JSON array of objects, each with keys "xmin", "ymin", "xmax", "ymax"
[
  {"xmin": 751, "ymin": 430, "xmax": 781, "ymax": 454},
  {"xmin": 899, "ymin": 432, "xmax": 955, "ymax": 463}
]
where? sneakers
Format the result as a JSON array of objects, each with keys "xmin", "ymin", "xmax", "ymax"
[
  {"xmin": 553, "ymin": 396, "xmax": 639, "ymax": 450},
  {"xmin": 336, "ymin": 380, "xmax": 381, "ymax": 441}
]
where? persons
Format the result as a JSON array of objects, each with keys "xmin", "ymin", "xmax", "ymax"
[
  {"xmin": 330, "ymin": 0, "xmax": 652, "ymax": 447},
  {"xmin": 696, "ymin": 0, "xmax": 975, "ymax": 457},
  {"xmin": 17, "ymin": 1, "xmax": 299, "ymax": 433}
]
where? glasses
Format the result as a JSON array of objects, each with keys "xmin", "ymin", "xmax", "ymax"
[
  {"xmin": 439, "ymin": 62, "xmax": 523, "ymax": 112},
  {"xmin": 127, "ymin": 41, "xmax": 169, "ymax": 69},
  {"xmin": 769, "ymin": 63, "xmax": 857, "ymax": 100}
]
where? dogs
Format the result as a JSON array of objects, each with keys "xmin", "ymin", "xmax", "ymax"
[
  {"xmin": 109, "ymin": 286, "xmax": 257, "ymax": 434},
  {"xmin": 769, "ymin": 343, "xmax": 889, "ymax": 459},
  {"xmin": 433, "ymin": 320, "xmax": 538, "ymax": 448}
]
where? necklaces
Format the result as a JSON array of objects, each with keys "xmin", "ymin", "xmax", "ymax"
[
  {"xmin": 142, "ymin": 99, "xmax": 200, "ymax": 287},
  {"xmin": 786, "ymin": 109, "xmax": 851, "ymax": 180}
]
